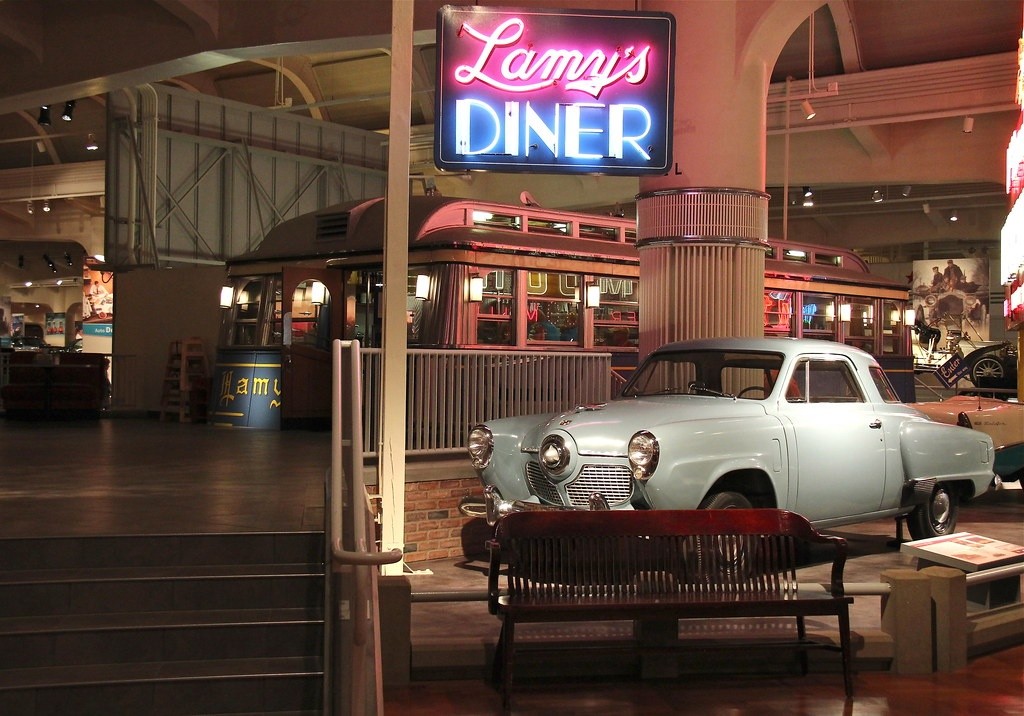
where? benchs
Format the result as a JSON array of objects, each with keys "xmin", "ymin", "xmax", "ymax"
[{"xmin": 489, "ymin": 507, "xmax": 854, "ymax": 716}]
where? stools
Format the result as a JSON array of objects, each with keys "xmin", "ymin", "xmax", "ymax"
[{"xmin": 158, "ymin": 338, "xmax": 210, "ymax": 424}]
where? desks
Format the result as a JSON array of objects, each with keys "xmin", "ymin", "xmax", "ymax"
[{"xmin": 899, "ymin": 531, "xmax": 1024, "ymax": 611}]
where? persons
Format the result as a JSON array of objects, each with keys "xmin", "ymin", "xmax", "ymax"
[
  {"xmin": 560, "ymin": 315, "xmax": 577, "ymax": 341},
  {"xmin": 931, "ymin": 260, "xmax": 962, "ymax": 293},
  {"xmin": 603, "ymin": 327, "xmax": 631, "ymax": 345},
  {"xmin": 530, "ymin": 303, "xmax": 561, "ymax": 341},
  {"xmin": 477, "ymin": 322, "xmax": 512, "ymax": 343},
  {"xmin": 90, "ymin": 281, "xmax": 107, "ymax": 295}
]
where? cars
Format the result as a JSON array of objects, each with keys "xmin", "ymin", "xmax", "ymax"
[
  {"xmin": 459, "ymin": 336, "xmax": 1004, "ymax": 587},
  {"xmin": 962, "ymin": 342, "xmax": 1016, "ymax": 390}
]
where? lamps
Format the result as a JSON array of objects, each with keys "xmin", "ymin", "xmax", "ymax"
[
  {"xmin": 922, "ymin": 201, "xmax": 930, "ymax": 214},
  {"xmin": 902, "ymin": 309, "xmax": 915, "ymax": 326},
  {"xmin": 902, "ymin": 186, "xmax": 911, "ymax": 196},
  {"xmin": 840, "ymin": 301, "xmax": 851, "ymax": 322},
  {"xmin": 37, "ymin": 106, "xmax": 50, "ymax": 127},
  {"xmin": 86, "ymin": 133, "xmax": 98, "ymax": 151},
  {"xmin": 963, "ymin": 115, "xmax": 975, "ymax": 133},
  {"xmin": 825, "ymin": 304, "xmax": 834, "ymax": 321},
  {"xmin": 218, "ymin": 285, "xmax": 235, "ymax": 309},
  {"xmin": 415, "ymin": 274, "xmax": 430, "ymax": 301},
  {"xmin": 891, "ymin": 309, "xmax": 901, "ymax": 322},
  {"xmin": 469, "ymin": 273, "xmax": 484, "ymax": 302},
  {"xmin": 872, "ymin": 190, "xmax": 883, "ymax": 203},
  {"xmin": 586, "ymin": 281, "xmax": 600, "ymax": 308},
  {"xmin": 800, "ymin": 99, "xmax": 815, "ymax": 120},
  {"xmin": 802, "ymin": 187, "xmax": 814, "ymax": 206},
  {"xmin": 950, "ymin": 210, "xmax": 958, "ymax": 221},
  {"xmin": 19, "ymin": 252, "xmax": 73, "ymax": 273},
  {"xmin": 311, "ymin": 282, "xmax": 327, "ymax": 306},
  {"xmin": 62, "ymin": 100, "xmax": 75, "ymax": 121},
  {"xmin": 27, "ymin": 200, "xmax": 50, "ymax": 215}
]
